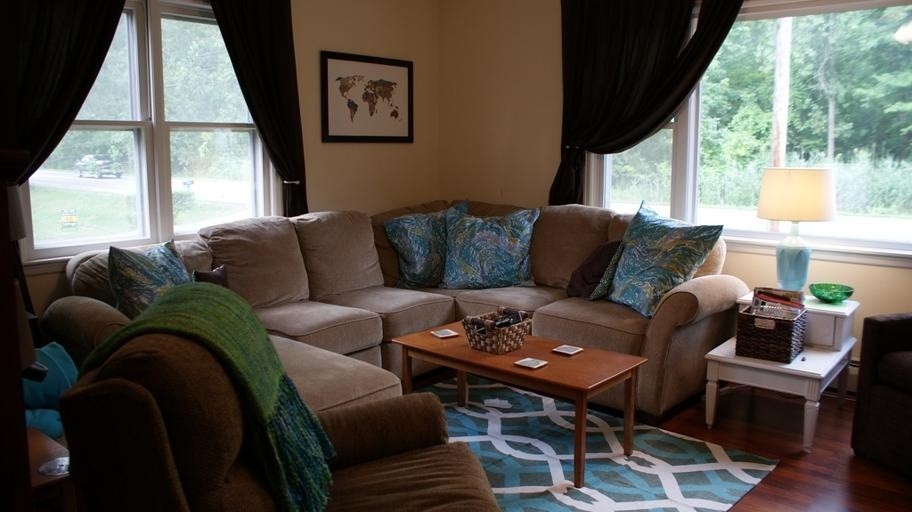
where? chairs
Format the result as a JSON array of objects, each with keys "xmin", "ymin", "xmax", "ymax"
[
  {"xmin": 849, "ymin": 312, "xmax": 911, "ymax": 479},
  {"xmin": 56, "ymin": 284, "xmax": 504, "ymax": 512}
]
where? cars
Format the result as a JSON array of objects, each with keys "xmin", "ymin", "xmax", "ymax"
[{"xmin": 75, "ymin": 152, "xmax": 123, "ymax": 180}]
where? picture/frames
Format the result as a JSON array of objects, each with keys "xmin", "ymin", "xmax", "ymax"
[{"xmin": 317, "ymin": 48, "xmax": 416, "ymax": 145}]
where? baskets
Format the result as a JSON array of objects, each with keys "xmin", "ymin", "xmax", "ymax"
[{"xmin": 460, "ymin": 304, "xmax": 533, "ymax": 356}]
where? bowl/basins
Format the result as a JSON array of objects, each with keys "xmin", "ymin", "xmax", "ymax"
[{"xmin": 808, "ymin": 283, "xmax": 854, "ymax": 303}]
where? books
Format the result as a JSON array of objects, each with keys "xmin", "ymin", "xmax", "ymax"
[{"xmin": 750, "ymin": 284, "xmax": 805, "ymax": 330}]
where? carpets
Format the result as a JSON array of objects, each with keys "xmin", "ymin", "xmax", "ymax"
[{"xmin": 401, "ymin": 369, "xmax": 779, "ymax": 512}]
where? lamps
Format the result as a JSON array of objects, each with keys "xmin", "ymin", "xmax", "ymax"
[{"xmin": 756, "ymin": 166, "xmax": 838, "ymax": 290}]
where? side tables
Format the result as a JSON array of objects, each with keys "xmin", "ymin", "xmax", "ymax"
[{"xmin": 705, "ymin": 289, "xmax": 861, "ymax": 452}]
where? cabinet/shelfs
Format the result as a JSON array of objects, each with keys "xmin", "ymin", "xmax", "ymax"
[{"xmin": 28, "ymin": 426, "xmax": 74, "ymax": 512}]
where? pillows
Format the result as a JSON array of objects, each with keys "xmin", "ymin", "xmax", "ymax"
[
  {"xmin": 108, "ymin": 240, "xmax": 191, "ymax": 322},
  {"xmin": 384, "ymin": 198, "xmax": 468, "ymax": 290},
  {"xmin": 607, "ymin": 200, "xmax": 724, "ymax": 319},
  {"xmin": 567, "ymin": 241, "xmax": 621, "ymax": 297},
  {"xmin": 190, "ymin": 264, "xmax": 230, "ymax": 287},
  {"xmin": 438, "ymin": 206, "xmax": 541, "ymax": 291}
]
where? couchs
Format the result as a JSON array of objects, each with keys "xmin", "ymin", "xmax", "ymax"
[{"xmin": 55, "ymin": 199, "xmax": 754, "ymax": 417}]
